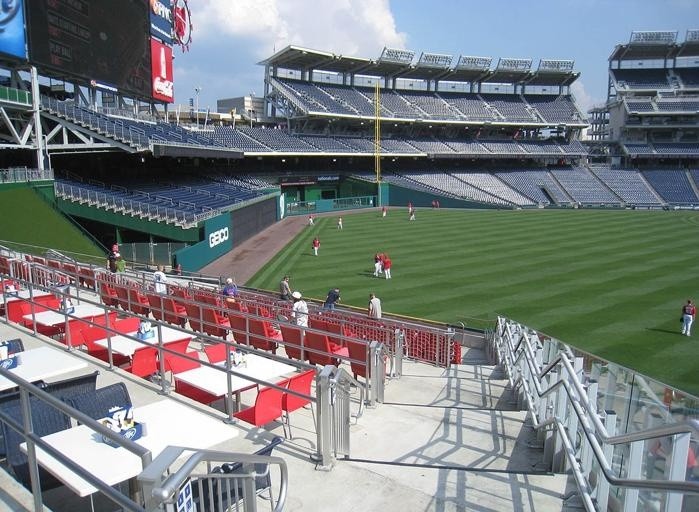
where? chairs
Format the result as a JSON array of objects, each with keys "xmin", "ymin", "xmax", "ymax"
[{"xmin": 0, "ymin": 254, "xmax": 376, "ymax": 512}]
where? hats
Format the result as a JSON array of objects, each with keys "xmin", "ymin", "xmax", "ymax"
[
  {"xmin": 226, "ymin": 278, "xmax": 232, "ymax": 284},
  {"xmin": 292, "ymin": 291, "xmax": 302, "ymax": 299}
]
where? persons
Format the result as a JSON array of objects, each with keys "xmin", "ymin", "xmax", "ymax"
[
  {"xmin": 431, "ymin": 199, "xmax": 436, "ymax": 209},
  {"xmin": 381, "ymin": 205, "xmax": 388, "ymax": 218},
  {"xmin": 307, "ymin": 213, "xmax": 316, "ymax": 226},
  {"xmin": 175, "ymin": 262, "xmax": 182, "ymax": 274},
  {"xmin": 382, "ymin": 255, "xmax": 392, "ymax": 280},
  {"xmin": 321, "ymin": 288, "xmax": 342, "ymax": 312},
  {"xmin": 335, "ymin": 215, "xmax": 345, "ymax": 230},
  {"xmin": 279, "ymin": 275, "xmax": 291, "ymax": 301},
  {"xmin": 406, "ymin": 201, "xmax": 414, "ymax": 215},
  {"xmin": 380, "ymin": 252, "xmax": 386, "ymax": 271},
  {"xmin": 114, "ymin": 255, "xmax": 126, "ymax": 274},
  {"xmin": 368, "ymin": 198, "xmax": 374, "ymax": 208},
  {"xmin": 409, "ymin": 207, "xmax": 417, "ymax": 221},
  {"xmin": 153, "ymin": 264, "xmax": 168, "ymax": 295},
  {"xmin": 312, "ymin": 235, "xmax": 321, "ymax": 257},
  {"xmin": 436, "ymin": 199, "xmax": 441, "ymax": 208},
  {"xmin": 679, "ymin": 297, "xmax": 696, "ymax": 338},
  {"xmin": 171, "ymin": 253, "xmax": 178, "ymax": 269},
  {"xmin": 222, "ymin": 276, "xmax": 241, "ymax": 300},
  {"xmin": 290, "ymin": 290, "xmax": 309, "ymax": 337},
  {"xmin": 367, "ymin": 291, "xmax": 382, "ymax": 323},
  {"xmin": 106, "ymin": 243, "xmax": 120, "ymax": 274},
  {"xmin": 373, "ymin": 252, "xmax": 380, "ymax": 278}
]
